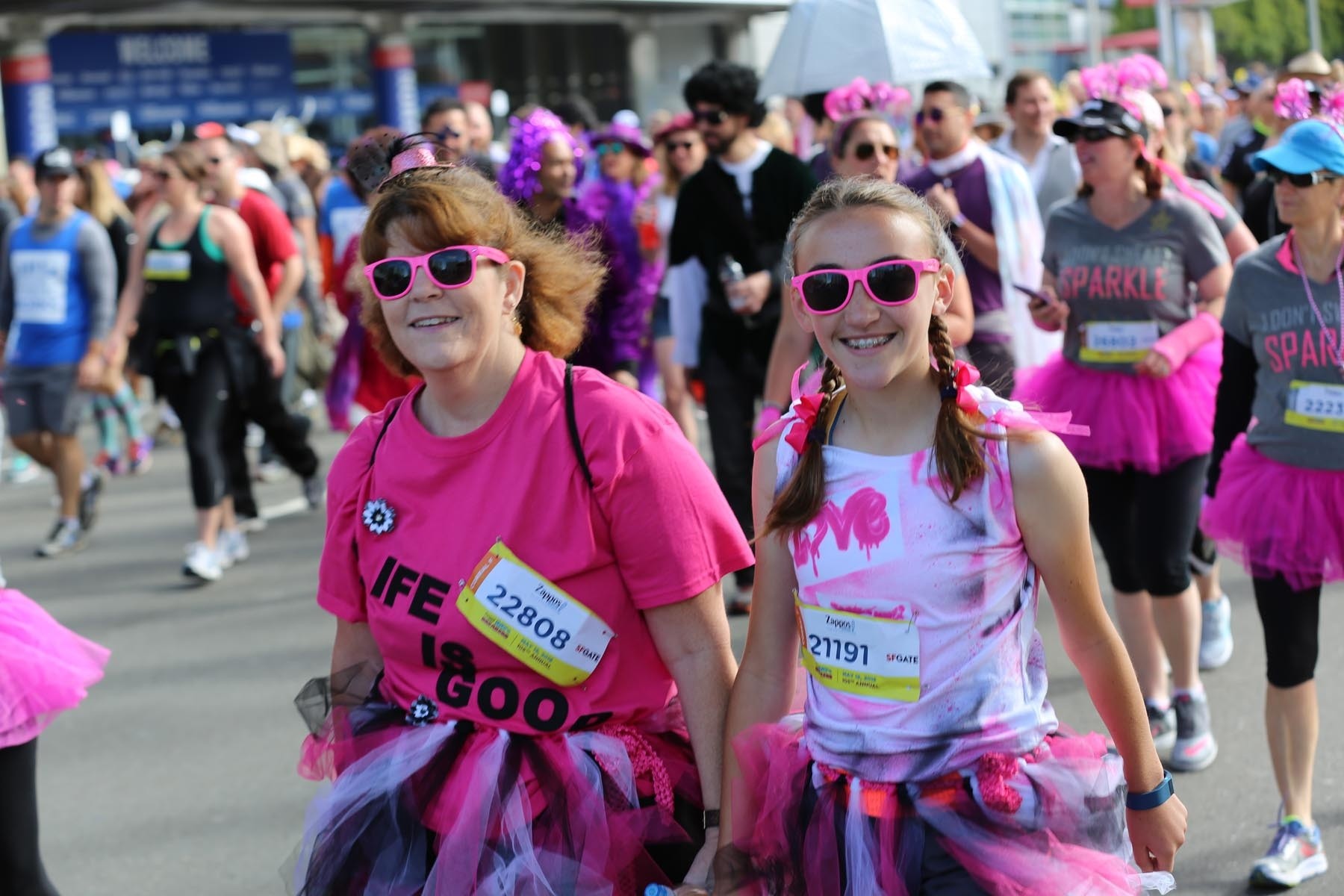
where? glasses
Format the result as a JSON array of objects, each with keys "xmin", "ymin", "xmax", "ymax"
[
  {"xmin": 691, "ymin": 109, "xmax": 730, "ymax": 125},
  {"xmin": 150, "ymin": 170, "xmax": 182, "ymax": 181},
  {"xmin": 364, "ymin": 246, "xmax": 512, "ymax": 301},
  {"xmin": 598, "ymin": 142, "xmax": 631, "ymax": 155},
  {"xmin": 200, "ymin": 156, "xmax": 238, "ymax": 166},
  {"xmin": 1158, "ymin": 104, "xmax": 1183, "ymax": 117},
  {"xmin": 1266, "ymin": 168, "xmax": 1336, "ymax": 186},
  {"xmin": 916, "ymin": 106, "xmax": 967, "ymax": 125},
  {"xmin": 842, "ymin": 141, "xmax": 901, "ymax": 164},
  {"xmin": 1065, "ymin": 128, "xmax": 1128, "ymax": 142},
  {"xmin": 1258, "ymin": 95, "xmax": 1275, "ymax": 102},
  {"xmin": 667, "ymin": 140, "xmax": 700, "ymax": 148},
  {"xmin": 790, "ymin": 259, "xmax": 940, "ymax": 315}
]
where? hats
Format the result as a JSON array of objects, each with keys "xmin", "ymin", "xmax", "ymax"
[
  {"xmin": 1289, "ymin": 50, "xmax": 1334, "ymax": 76},
  {"xmin": 684, "ymin": 61, "xmax": 767, "ymax": 127},
  {"xmin": 1052, "ymin": 98, "xmax": 1146, "ymax": 139},
  {"xmin": 1253, "ymin": 118, "xmax": 1344, "ymax": 177},
  {"xmin": 33, "ymin": 146, "xmax": 78, "ymax": 176},
  {"xmin": 591, "ymin": 124, "xmax": 654, "ymax": 157},
  {"xmin": 652, "ymin": 114, "xmax": 698, "ymax": 146}
]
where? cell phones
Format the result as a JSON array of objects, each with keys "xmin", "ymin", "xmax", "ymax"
[{"xmin": 1014, "ymin": 284, "xmax": 1054, "ymax": 307}]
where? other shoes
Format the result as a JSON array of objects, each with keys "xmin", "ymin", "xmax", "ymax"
[{"xmin": 7, "ymin": 417, "xmax": 326, "ymax": 528}]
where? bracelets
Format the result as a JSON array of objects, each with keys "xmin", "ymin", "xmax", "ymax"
[
  {"xmin": 1125, "ymin": 768, "xmax": 1175, "ymax": 812},
  {"xmin": 703, "ymin": 808, "xmax": 723, "ymax": 832}
]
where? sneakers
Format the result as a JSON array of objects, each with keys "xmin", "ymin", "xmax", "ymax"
[
  {"xmin": 40, "ymin": 518, "xmax": 82, "ymax": 558},
  {"xmin": 1168, "ymin": 688, "xmax": 1219, "ymax": 770},
  {"xmin": 213, "ymin": 528, "xmax": 251, "ymax": 567},
  {"xmin": 1193, "ymin": 593, "xmax": 1234, "ymax": 671},
  {"xmin": 1248, "ymin": 818, "xmax": 1327, "ymax": 891},
  {"xmin": 183, "ymin": 542, "xmax": 224, "ymax": 581},
  {"xmin": 730, "ymin": 584, "xmax": 753, "ymax": 614},
  {"xmin": 1107, "ymin": 706, "xmax": 1175, "ymax": 758}
]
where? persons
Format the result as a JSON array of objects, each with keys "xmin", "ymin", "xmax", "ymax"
[
  {"xmin": 287, "ymin": 131, "xmax": 758, "ymax": 894},
  {"xmin": 703, "ymin": 171, "xmax": 1191, "ymax": 896},
  {"xmin": 1103, "ymin": 82, "xmax": 1265, "ymax": 673},
  {"xmin": 1003, "ymin": 88, "xmax": 1234, "ymax": 776},
  {"xmin": 1199, "ymin": 113, "xmax": 1341, "ymax": 891},
  {"xmin": 0, "ymin": 567, "xmax": 114, "ymax": 896},
  {"xmin": 2, "ymin": 47, "xmax": 1344, "ymax": 585}
]
